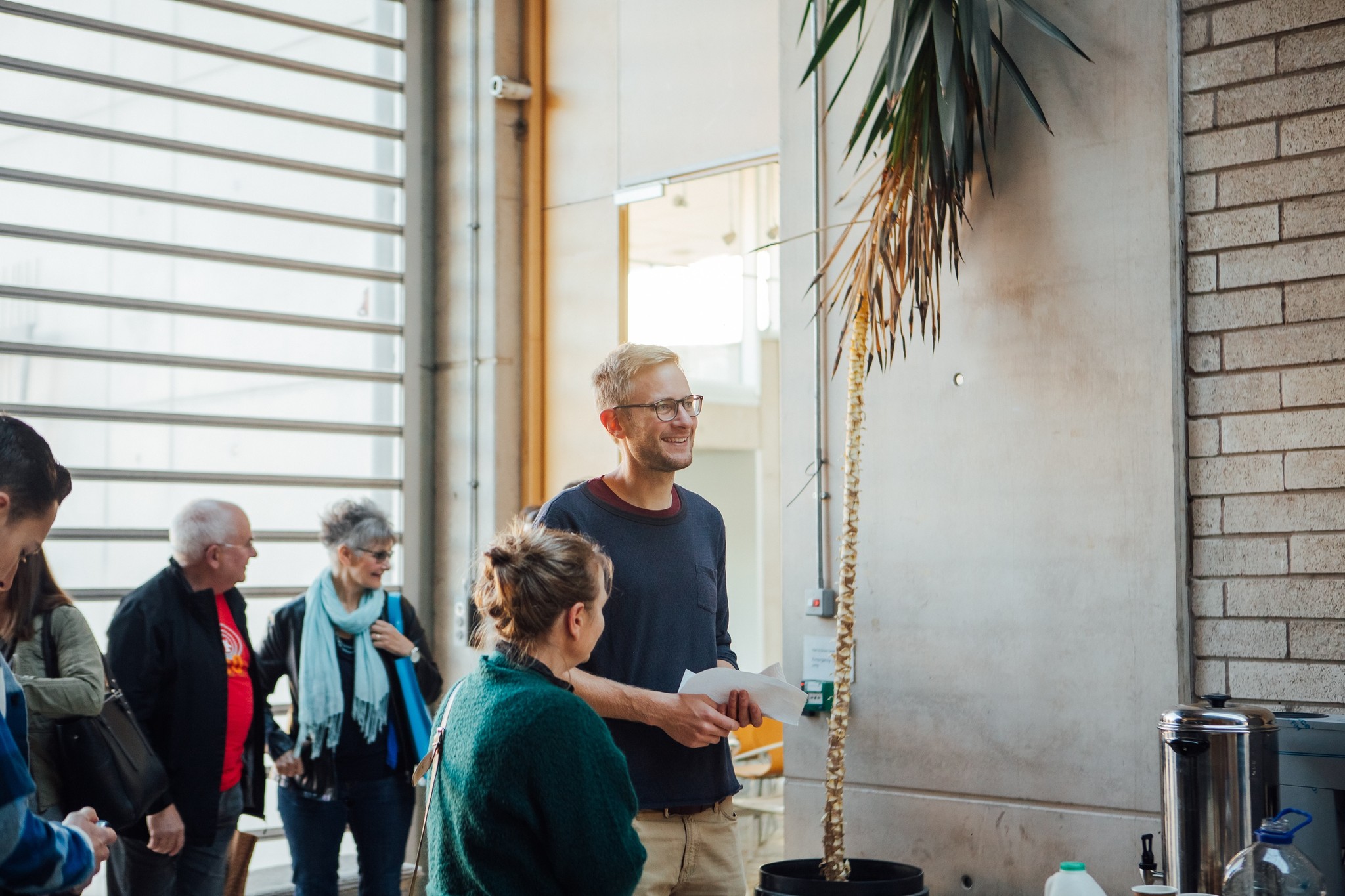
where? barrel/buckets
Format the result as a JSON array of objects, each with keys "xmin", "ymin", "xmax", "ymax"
[{"xmin": 1139, "ymin": 691, "xmax": 1281, "ymax": 896}]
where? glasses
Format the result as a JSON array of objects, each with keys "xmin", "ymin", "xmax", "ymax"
[
  {"xmin": 204, "ymin": 540, "xmax": 253, "ymax": 552},
  {"xmin": 352, "ymin": 546, "xmax": 393, "ymax": 563},
  {"xmin": 612, "ymin": 394, "xmax": 704, "ymax": 422}
]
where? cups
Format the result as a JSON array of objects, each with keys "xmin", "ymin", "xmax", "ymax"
[
  {"xmin": 1131, "ymin": 885, "xmax": 1179, "ymax": 896},
  {"xmin": 1177, "ymin": 893, "xmax": 1219, "ymax": 896}
]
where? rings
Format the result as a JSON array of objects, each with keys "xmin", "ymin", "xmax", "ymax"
[{"xmin": 375, "ymin": 633, "xmax": 381, "ymax": 642}]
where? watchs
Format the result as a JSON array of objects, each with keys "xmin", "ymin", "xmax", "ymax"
[{"xmin": 409, "ymin": 647, "xmax": 422, "ymax": 663}]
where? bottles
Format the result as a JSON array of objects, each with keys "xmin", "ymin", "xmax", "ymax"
[
  {"xmin": 1221, "ymin": 806, "xmax": 1328, "ymax": 896},
  {"xmin": 1043, "ymin": 861, "xmax": 1107, "ymax": 896}
]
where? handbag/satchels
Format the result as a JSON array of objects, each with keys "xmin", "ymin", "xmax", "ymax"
[{"xmin": 38, "ymin": 606, "xmax": 169, "ymax": 834}]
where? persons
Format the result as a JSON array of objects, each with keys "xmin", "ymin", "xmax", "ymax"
[
  {"xmin": 258, "ymin": 497, "xmax": 444, "ymax": 896},
  {"xmin": 425, "ymin": 513, "xmax": 648, "ymax": 896},
  {"xmin": 106, "ymin": 499, "xmax": 269, "ymax": 896},
  {"xmin": 0, "ymin": 414, "xmax": 118, "ymax": 896},
  {"xmin": 529, "ymin": 343, "xmax": 762, "ymax": 896}
]
